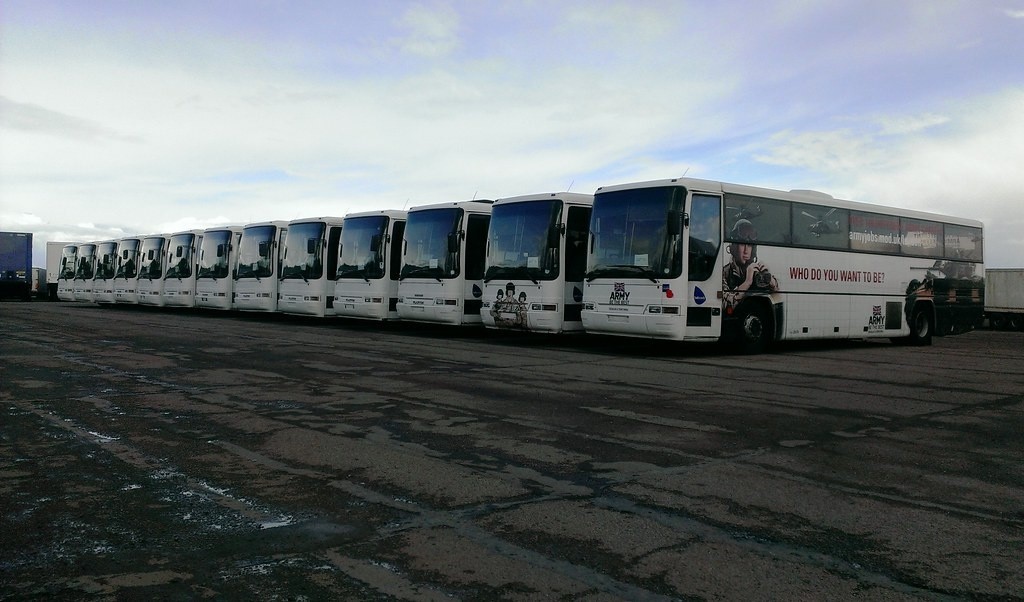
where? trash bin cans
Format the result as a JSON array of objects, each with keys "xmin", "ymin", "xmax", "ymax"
[{"xmin": 47, "ymin": 283, "xmax": 58, "ymax": 302}]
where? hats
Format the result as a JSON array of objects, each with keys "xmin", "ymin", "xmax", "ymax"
[
  {"xmin": 496, "ymin": 289, "xmax": 504, "ymax": 299},
  {"xmin": 519, "ymin": 292, "xmax": 527, "ymax": 302},
  {"xmin": 506, "ymin": 283, "xmax": 515, "ymax": 296}
]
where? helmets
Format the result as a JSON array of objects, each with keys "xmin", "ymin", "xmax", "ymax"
[{"xmin": 731, "ymin": 219, "xmax": 758, "ymax": 242}]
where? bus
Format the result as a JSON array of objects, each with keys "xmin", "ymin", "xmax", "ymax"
[
  {"xmin": 232, "ymin": 218, "xmax": 294, "ymax": 314},
  {"xmin": 194, "ymin": 225, "xmax": 245, "ymax": 311},
  {"xmin": 135, "ymin": 233, "xmax": 163, "ymax": 307},
  {"xmin": 277, "ymin": 216, "xmax": 355, "ymax": 318},
  {"xmin": 56, "ymin": 242, "xmax": 83, "ymax": 302},
  {"xmin": 332, "ymin": 210, "xmax": 419, "ymax": 323},
  {"xmin": 112, "ymin": 236, "xmax": 142, "ymax": 305},
  {"xmin": 73, "ymin": 241, "xmax": 100, "ymax": 302},
  {"xmin": 396, "ymin": 199, "xmax": 498, "ymax": 327},
  {"xmin": 162, "ymin": 228, "xmax": 206, "ymax": 308},
  {"xmin": 480, "ymin": 190, "xmax": 620, "ymax": 333},
  {"xmin": 91, "ymin": 239, "xmax": 119, "ymax": 304},
  {"xmin": 581, "ymin": 175, "xmax": 985, "ymax": 357}
]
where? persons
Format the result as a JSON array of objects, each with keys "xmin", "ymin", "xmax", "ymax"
[{"xmin": 722, "ymin": 219, "xmax": 778, "ymax": 307}]
